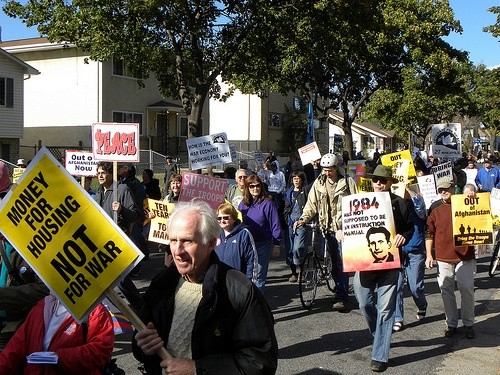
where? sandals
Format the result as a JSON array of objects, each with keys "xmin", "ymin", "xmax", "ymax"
[
  {"xmin": 416, "ymin": 308, "xmax": 426, "ymax": 320},
  {"xmin": 392, "ymin": 321, "xmax": 403, "ymax": 331}
]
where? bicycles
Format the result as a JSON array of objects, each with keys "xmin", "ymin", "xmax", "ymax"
[
  {"xmin": 488, "ymin": 242, "xmax": 500, "ymax": 277},
  {"xmin": 289, "ymin": 222, "xmax": 339, "ymax": 310}
]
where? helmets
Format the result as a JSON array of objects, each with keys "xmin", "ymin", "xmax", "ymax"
[{"xmin": 319, "ymin": 153, "xmax": 339, "ymax": 167}]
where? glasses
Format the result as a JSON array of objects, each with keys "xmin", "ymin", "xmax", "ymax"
[
  {"xmin": 236, "ymin": 176, "xmax": 248, "ymax": 179},
  {"xmin": 372, "ymin": 177, "xmax": 389, "ymax": 184},
  {"xmin": 96, "ymin": 171, "xmax": 109, "ymax": 175},
  {"xmin": 217, "ymin": 216, "xmax": 232, "ymax": 220},
  {"xmin": 249, "ymin": 184, "xmax": 261, "ymax": 188}
]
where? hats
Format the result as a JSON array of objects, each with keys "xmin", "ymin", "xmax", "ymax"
[
  {"xmin": 366, "ymin": 165, "xmax": 398, "ymax": 181},
  {"xmin": 484, "ymin": 158, "xmax": 493, "ymax": 163},
  {"xmin": 436, "ymin": 176, "xmax": 454, "ymax": 189}
]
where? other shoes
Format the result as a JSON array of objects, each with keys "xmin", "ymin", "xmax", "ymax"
[
  {"xmin": 371, "ymin": 359, "xmax": 386, "ymax": 371},
  {"xmin": 332, "ymin": 301, "xmax": 345, "ymax": 309},
  {"xmin": 445, "ymin": 325, "xmax": 454, "ymax": 337},
  {"xmin": 288, "ymin": 274, "xmax": 297, "ymax": 281},
  {"xmin": 464, "ymin": 324, "xmax": 474, "ymax": 338},
  {"xmin": 298, "ymin": 274, "xmax": 306, "ymax": 282}
]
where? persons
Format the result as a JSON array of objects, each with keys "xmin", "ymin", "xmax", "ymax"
[
  {"xmin": 238, "ymin": 174, "xmax": 281, "ymax": 294},
  {"xmin": 0, "ymin": 235, "xmax": 50, "ymax": 326},
  {"xmin": 116, "ymin": 162, "xmax": 147, "ymax": 279},
  {"xmin": 390, "ymin": 180, "xmax": 426, "ymax": 332},
  {"xmin": 336, "ymin": 164, "xmax": 417, "ymax": 371},
  {"xmin": 162, "ymin": 155, "xmax": 178, "ymax": 197},
  {"xmin": 355, "ymin": 145, "xmax": 500, "ymax": 233},
  {"xmin": 282, "ymin": 172, "xmax": 314, "ymax": 284},
  {"xmin": 0, "ymin": 161, "xmax": 16, "ymax": 205},
  {"xmin": 94, "ymin": 162, "xmax": 145, "ymax": 309},
  {"xmin": 132, "ymin": 196, "xmax": 278, "ymax": 374},
  {"xmin": 17, "ymin": 158, "xmax": 28, "ymax": 169},
  {"xmin": 148, "ymin": 172, "xmax": 184, "ymax": 272},
  {"xmin": 214, "ymin": 203, "xmax": 259, "ymax": 289},
  {"xmin": 206, "ymin": 155, "xmax": 323, "ymax": 256},
  {"xmin": 139, "ymin": 167, "xmax": 163, "ymax": 204},
  {"xmin": 293, "ymin": 153, "xmax": 361, "ymax": 308},
  {"xmin": 0, "ymin": 284, "xmax": 115, "ymax": 375},
  {"xmin": 425, "ymin": 177, "xmax": 480, "ymax": 338}
]
